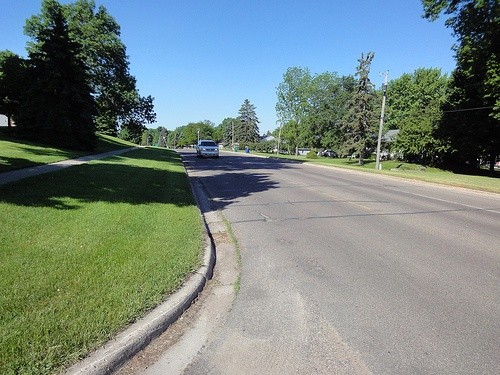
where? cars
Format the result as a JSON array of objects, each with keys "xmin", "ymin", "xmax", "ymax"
[{"xmin": 196, "ymin": 140, "xmax": 219, "ymax": 159}]
